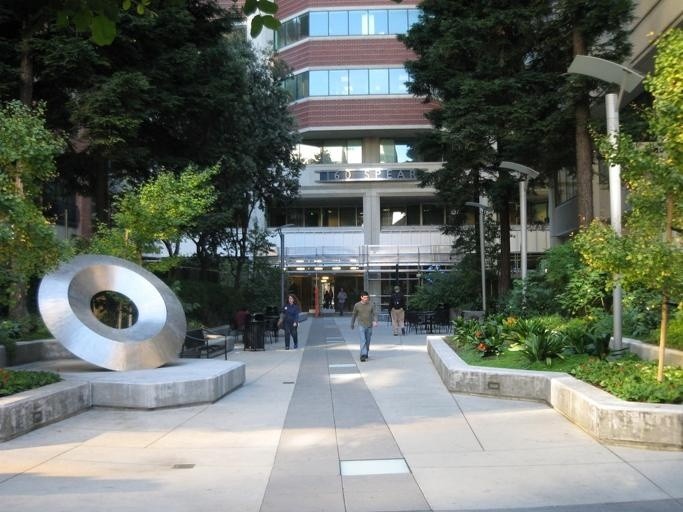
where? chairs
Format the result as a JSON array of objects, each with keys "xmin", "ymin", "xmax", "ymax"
[
  {"xmin": 229, "ymin": 313, "xmax": 280, "ymax": 344},
  {"xmin": 404, "ymin": 308, "xmax": 452, "ymax": 334}
]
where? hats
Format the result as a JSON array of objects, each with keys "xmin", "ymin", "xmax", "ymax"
[{"xmin": 394, "ymin": 286, "xmax": 400, "ymax": 293}]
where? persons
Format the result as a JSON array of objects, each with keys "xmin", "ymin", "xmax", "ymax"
[
  {"xmin": 337, "ymin": 287, "xmax": 348, "ymax": 316},
  {"xmin": 234, "ymin": 305, "xmax": 250, "ymax": 330},
  {"xmin": 350, "ymin": 291, "xmax": 376, "ymax": 362},
  {"xmin": 277, "ymin": 294, "xmax": 300, "ymax": 350},
  {"xmin": 389, "ymin": 285, "xmax": 406, "ymax": 335},
  {"xmin": 324, "ymin": 287, "xmax": 333, "ymax": 308}
]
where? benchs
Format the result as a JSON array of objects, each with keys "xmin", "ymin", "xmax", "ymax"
[{"xmin": 180, "ymin": 327, "xmax": 227, "ymax": 360}]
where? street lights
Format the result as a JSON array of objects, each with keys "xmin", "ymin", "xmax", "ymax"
[
  {"xmin": 497, "ymin": 160, "xmax": 542, "ymax": 312},
  {"xmin": 270, "ymin": 223, "xmax": 296, "ymax": 312},
  {"xmin": 465, "ymin": 199, "xmax": 493, "ymax": 318},
  {"xmin": 566, "ymin": 53, "xmax": 643, "ymax": 355}
]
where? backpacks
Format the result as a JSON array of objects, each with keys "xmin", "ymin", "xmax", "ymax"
[{"xmin": 394, "ymin": 294, "xmax": 402, "ymax": 309}]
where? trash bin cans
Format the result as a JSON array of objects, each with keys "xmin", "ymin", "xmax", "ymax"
[{"xmin": 246, "ymin": 312, "xmax": 264, "ymax": 350}]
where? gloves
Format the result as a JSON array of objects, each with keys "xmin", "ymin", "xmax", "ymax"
[{"xmin": 293, "ymin": 322, "xmax": 297, "ymax": 327}]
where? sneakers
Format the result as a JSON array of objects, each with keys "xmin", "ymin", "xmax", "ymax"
[{"xmin": 360, "ymin": 355, "xmax": 368, "ymax": 362}]
